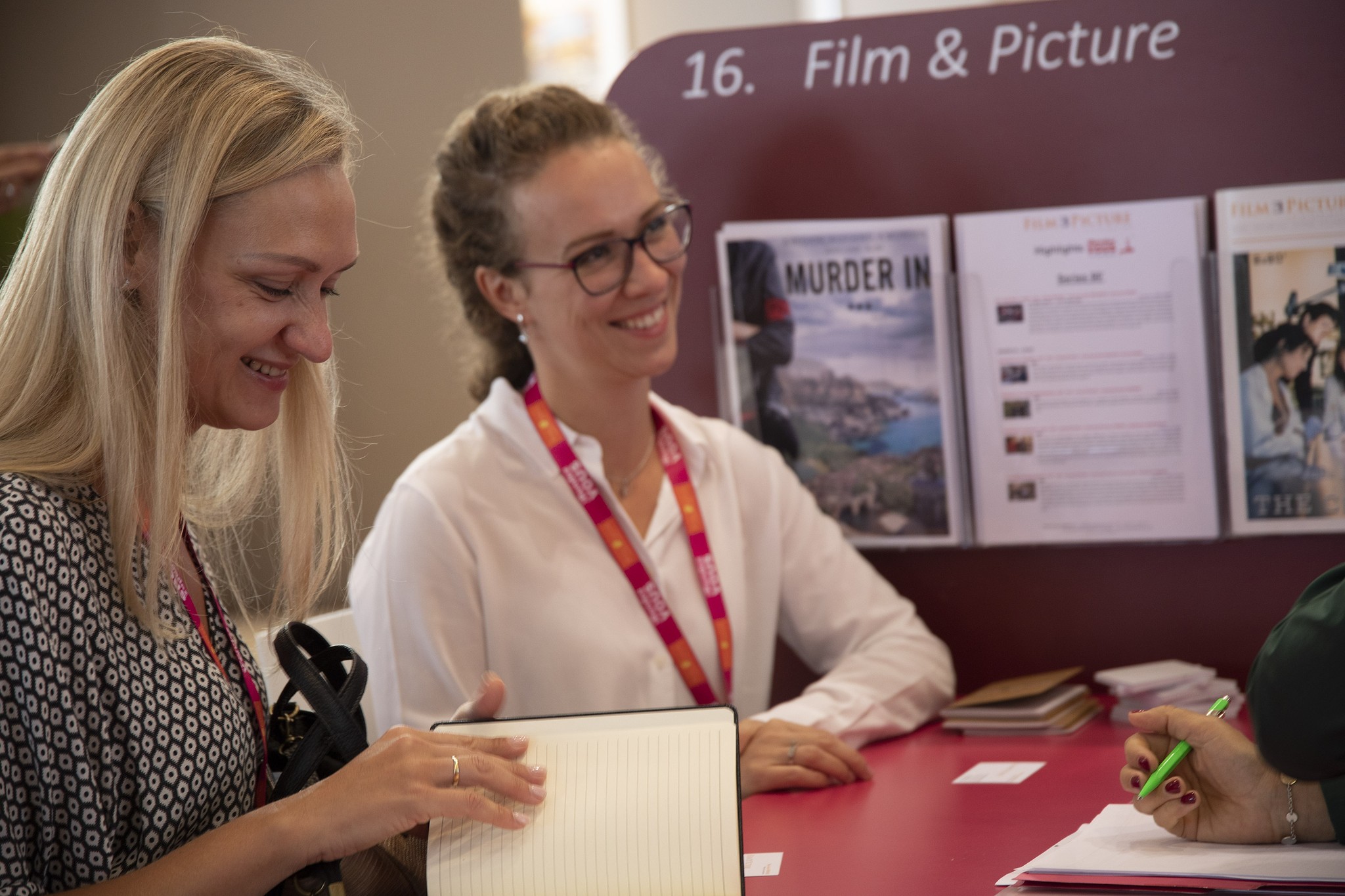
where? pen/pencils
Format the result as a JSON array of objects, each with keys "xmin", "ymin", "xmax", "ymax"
[{"xmin": 1136, "ymin": 694, "xmax": 1232, "ymax": 801}]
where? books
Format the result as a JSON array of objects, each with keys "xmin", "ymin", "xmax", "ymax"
[
  {"xmin": 937, "ymin": 663, "xmax": 1101, "ymax": 735},
  {"xmin": 1094, "ymin": 657, "xmax": 1249, "ymax": 728},
  {"xmin": 425, "ymin": 705, "xmax": 749, "ymax": 896},
  {"xmin": 1011, "ymin": 799, "xmax": 1343, "ymax": 896}
]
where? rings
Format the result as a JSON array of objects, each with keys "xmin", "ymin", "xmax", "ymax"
[{"xmin": 452, "ymin": 754, "xmax": 460, "ymax": 789}]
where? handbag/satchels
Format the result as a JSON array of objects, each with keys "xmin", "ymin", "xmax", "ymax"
[{"xmin": 258, "ymin": 701, "xmax": 429, "ymax": 896}]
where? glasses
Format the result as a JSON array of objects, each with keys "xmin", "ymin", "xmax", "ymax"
[{"xmin": 500, "ymin": 200, "xmax": 695, "ymax": 297}]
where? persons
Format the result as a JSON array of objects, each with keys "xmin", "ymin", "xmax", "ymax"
[
  {"xmin": 1237, "ymin": 318, "xmax": 1335, "ymax": 520},
  {"xmin": 1, "ymin": 38, "xmax": 549, "ymax": 895},
  {"xmin": 1275, "ymin": 300, "xmax": 1343, "ymax": 504},
  {"xmin": 1123, "ymin": 562, "xmax": 1344, "ymax": 851},
  {"xmin": 341, "ymin": 78, "xmax": 954, "ymax": 800},
  {"xmin": 725, "ymin": 238, "xmax": 801, "ymax": 469}
]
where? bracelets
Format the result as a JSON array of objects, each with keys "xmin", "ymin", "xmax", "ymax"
[{"xmin": 1276, "ymin": 769, "xmax": 1300, "ymax": 845}]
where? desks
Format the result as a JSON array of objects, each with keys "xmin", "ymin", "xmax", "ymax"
[{"xmin": 742, "ymin": 694, "xmax": 1256, "ymax": 896}]
